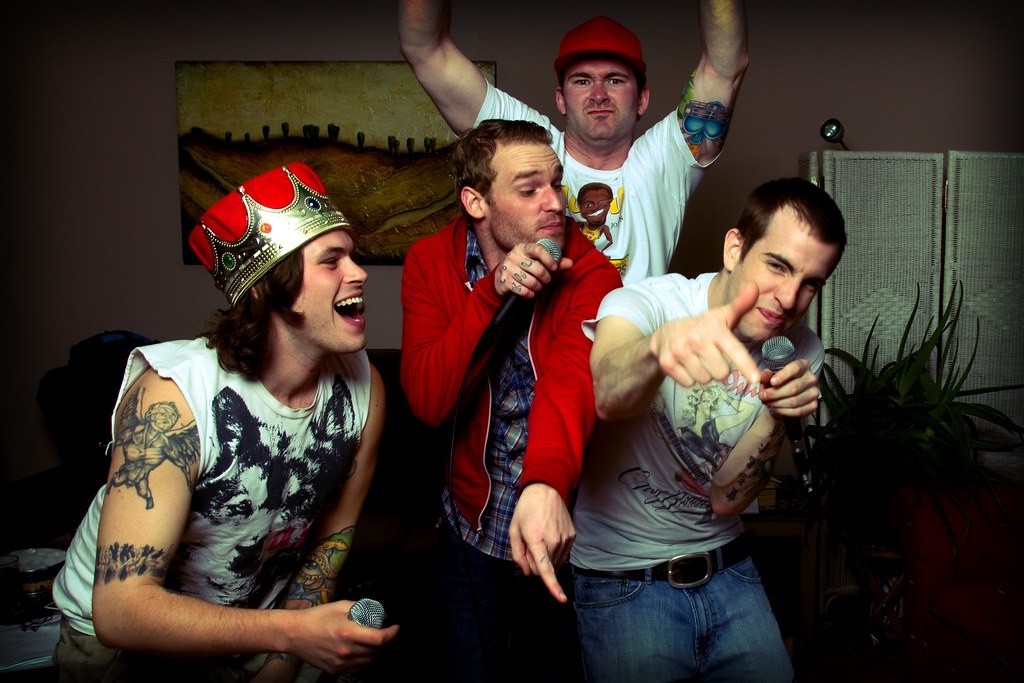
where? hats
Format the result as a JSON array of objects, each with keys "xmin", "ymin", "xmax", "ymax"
[
  {"xmin": 554, "ymin": 15, "xmax": 647, "ymax": 88},
  {"xmin": 189, "ymin": 161, "xmax": 352, "ymax": 309}
]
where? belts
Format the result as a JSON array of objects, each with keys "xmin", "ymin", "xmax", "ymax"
[{"xmin": 571, "ymin": 533, "xmax": 750, "ymax": 589}]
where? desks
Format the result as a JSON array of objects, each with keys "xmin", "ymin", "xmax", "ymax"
[{"xmin": 752, "ymin": 509, "xmax": 820, "ymax": 661}]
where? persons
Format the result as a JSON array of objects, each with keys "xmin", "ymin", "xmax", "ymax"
[
  {"xmin": 51, "ymin": 160, "xmax": 400, "ymax": 683},
  {"xmin": 568, "ymin": 176, "xmax": 846, "ymax": 683},
  {"xmin": 400, "ymin": 120, "xmax": 623, "ymax": 683},
  {"xmin": 396, "ymin": 0, "xmax": 749, "ymax": 288}
]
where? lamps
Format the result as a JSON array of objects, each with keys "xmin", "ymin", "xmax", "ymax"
[{"xmin": 819, "ymin": 118, "xmax": 850, "ymax": 151}]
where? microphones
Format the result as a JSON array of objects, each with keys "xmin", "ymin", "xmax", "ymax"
[
  {"xmin": 315, "ymin": 598, "xmax": 386, "ymax": 683},
  {"xmin": 761, "ymin": 336, "xmax": 806, "ymax": 473},
  {"xmin": 471, "ymin": 239, "xmax": 561, "ymax": 363}
]
